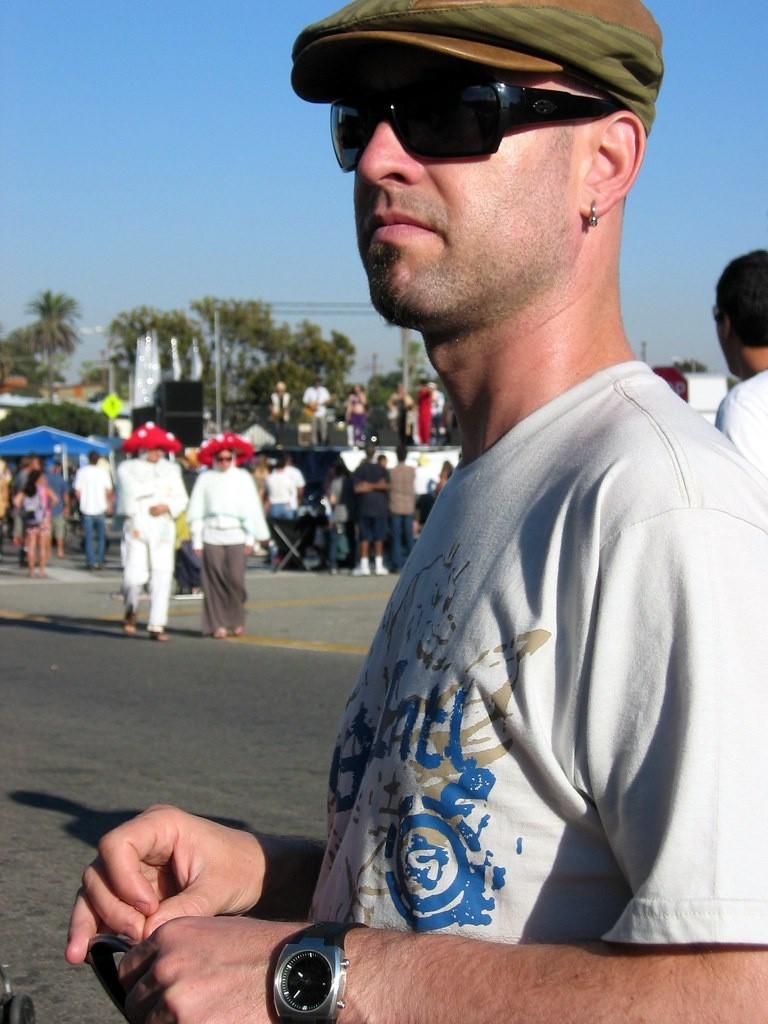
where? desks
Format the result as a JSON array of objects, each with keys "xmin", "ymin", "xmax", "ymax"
[{"xmin": 268, "ymin": 516, "xmax": 321, "ymax": 572}]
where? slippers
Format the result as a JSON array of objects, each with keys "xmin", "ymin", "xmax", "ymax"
[
  {"xmin": 234, "ymin": 626, "xmax": 245, "ymax": 634},
  {"xmin": 213, "ymin": 628, "xmax": 226, "ymax": 638}
]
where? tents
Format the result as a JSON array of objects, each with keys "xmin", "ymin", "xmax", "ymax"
[{"xmin": 0, "ymin": 425, "xmax": 126, "ymax": 456}]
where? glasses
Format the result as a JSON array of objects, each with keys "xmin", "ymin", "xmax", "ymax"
[
  {"xmin": 329, "ymin": 79, "xmax": 618, "ymax": 174},
  {"xmin": 216, "ymin": 456, "xmax": 233, "ymax": 462}
]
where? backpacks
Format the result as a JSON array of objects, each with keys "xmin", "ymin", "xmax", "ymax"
[{"xmin": 21, "ymin": 486, "xmax": 44, "ymax": 524}]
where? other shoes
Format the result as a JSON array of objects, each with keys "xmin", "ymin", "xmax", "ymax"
[
  {"xmin": 377, "ymin": 567, "xmax": 390, "ymax": 576},
  {"xmin": 351, "ymin": 566, "xmax": 369, "ymax": 576}
]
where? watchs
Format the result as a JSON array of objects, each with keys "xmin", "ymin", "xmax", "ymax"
[{"xmin": 272, "ymin": 922, "xmax": 368, "ymax": 1024}]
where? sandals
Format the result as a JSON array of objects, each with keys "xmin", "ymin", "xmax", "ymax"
[
  {"xmin": 124, "ymin": 618, "xmax": 136, "ymax": 635},
  {"xmin": 150, "ymin": 632, "xmax": 168, "ymax": 640}
]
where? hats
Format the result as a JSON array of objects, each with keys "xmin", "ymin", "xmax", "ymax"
[
  {"xmin": 196, "ymin": 433, "xmax": 254, "ymax": 465},
  {"xmin": 291, "ymin": 0, "xmax": 665, "ymax": 138},
  {"xmin": 44, "ymin": 458, "xmax": 56, "ymax": 471},
  {"xmin": 122, "ymin": 419, "xmax": 184, "ymax": 453}
]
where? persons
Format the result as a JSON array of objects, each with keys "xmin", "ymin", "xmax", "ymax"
[
  {"xmin": 115, "ymin": 421, "xmax": 188, "ymax": 641},
  {"xmin": 303, "ymin": 376, "xmax": 335, "ymax": 446},
  {"xmin": 714, "ymin": 249, "xmax": 768, "ymax": 474},
  {"xmin": 268, "ymin": 381, "xmax": 297, "ymax": 451},
  {"xmin": 66, "ymin": 0, "xmax": 768, "ymax": 1022},
  {"xmin": 386, "ymin": 381, "xmax": 445, "ymax": 446},
  {"xmin": 0, "ymin": 444, "xmax": 454, "ymax": 582},
  {"xmin": 345, "ymin": 384, "xmax": 367, "ymax": 446},
  {"xmin": 187, "ymin": 431, "xmax": 272, "ymax": 639}
]
